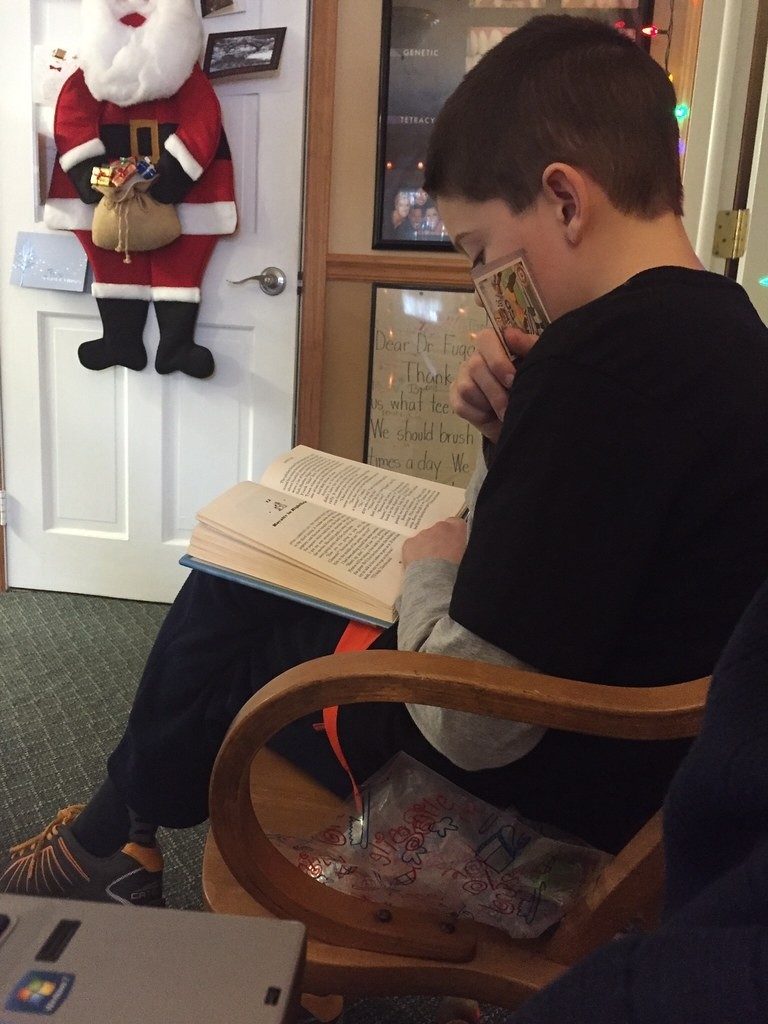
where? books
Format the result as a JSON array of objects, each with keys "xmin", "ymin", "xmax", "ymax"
[{"xmin": 178, "ymin": 444, "xmax": 470, "ymax": 628}]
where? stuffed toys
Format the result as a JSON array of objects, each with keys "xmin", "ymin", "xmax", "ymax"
[{"xmin": 43, "ymin": 0, "xmax": 238, "ymax": 380}]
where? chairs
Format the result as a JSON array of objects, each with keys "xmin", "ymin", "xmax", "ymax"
[{"xmin": 199, "ymin": 650, "xmax": 711, "ymax": 1024}]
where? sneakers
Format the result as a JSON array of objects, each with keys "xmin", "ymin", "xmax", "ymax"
[{"xmin": 0, "ymin": 801, "xmax": 167, "ymax": 913}]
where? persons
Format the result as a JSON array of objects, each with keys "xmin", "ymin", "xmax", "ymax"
[
  {"xmin": 392, "ymin": 189, "xmax": 449, "ymax": 235},
  {"xmin": 218, "ymin": 48, "xmax": 251, "ymax": 69},
  {"xmin": 0, "ymin": 14, "xmax": 767, "ymax": 907}
]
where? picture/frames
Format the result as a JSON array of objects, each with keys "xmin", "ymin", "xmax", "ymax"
[
  {"xmin": 371, "ymin": 0, "xmax": 657, "ymax": 253},
  {"xmin": 202, "ymin": 27, "xmax": 287, "ymax": 80}
]
